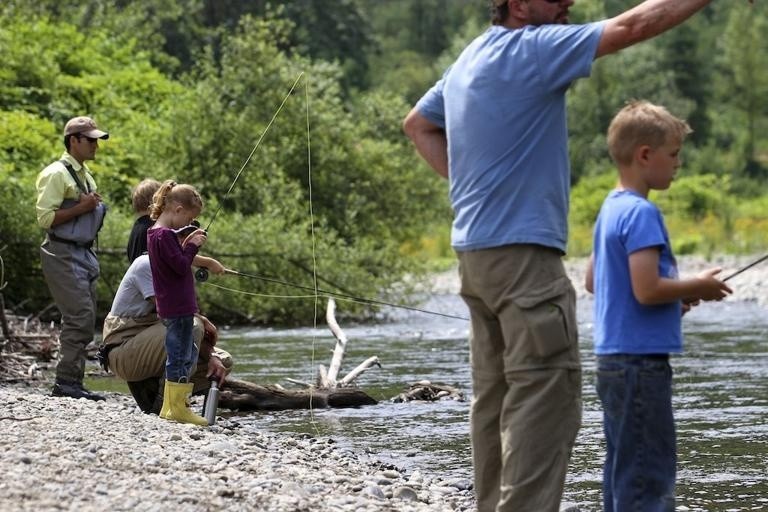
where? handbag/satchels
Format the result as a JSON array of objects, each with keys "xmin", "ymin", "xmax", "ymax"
[{"xmin": 45, "ymin": 159, "xmax": 104, "ymax": 244}]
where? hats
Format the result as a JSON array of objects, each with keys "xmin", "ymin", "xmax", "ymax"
[{"xmin": 63, "ymin": 117, "xmax": 109, "ymax": 141}]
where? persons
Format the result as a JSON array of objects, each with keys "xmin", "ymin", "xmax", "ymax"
[
  {"xmin": 402, "ymin": 0, "xmax": 713, "ymax": 512},
  {"xmin": 148, "ymin": 180, "xmax": 209, "ymax": 425},
  {"xmin": 587, "ymin": 99, "xmax": 735, "ymax": 511},
  {"xmin": 35, "ymin": 116, "xmax": 110, "ymax": 402},
  {"xmin": 96, "ymin": 226, "xmax": 233, "ymax": 416},
  {"xmin": 127, "ymin": 178, "xmax": 162, "ymax": 264}
]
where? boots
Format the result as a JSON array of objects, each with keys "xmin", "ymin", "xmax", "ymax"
[
  {"xmin": 165, "ymin": 382, "xmax": 209, "ymax": 427},
  {"xmin": 160, "ymin": 384, "xmax": 170, "ymax": 418}
]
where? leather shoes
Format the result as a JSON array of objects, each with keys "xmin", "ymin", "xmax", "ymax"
[{"xmin": 50, "ymin": 382, "xmax": 108, "ymax": 402}]
